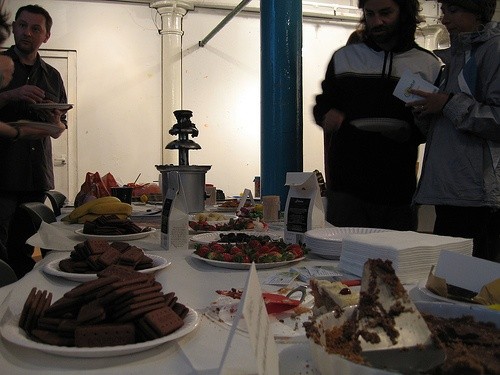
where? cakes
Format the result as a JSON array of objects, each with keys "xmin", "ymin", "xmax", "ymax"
[{"xmin": 308, "ymin": 279, "xmax": 361, "ymax": 319}]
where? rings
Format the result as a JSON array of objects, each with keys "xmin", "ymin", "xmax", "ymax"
[
  {"xmin": 30, "ymin": 93, "xmax": 33, "ymax": 97},
  {"xmin": 421, "ymin": 105, "xmax": 423, "ymax": 109}
]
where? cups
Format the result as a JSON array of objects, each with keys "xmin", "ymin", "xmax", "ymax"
[{"xmin": 111, "ymin": 187, "xmax": 133, "ymax": 205}]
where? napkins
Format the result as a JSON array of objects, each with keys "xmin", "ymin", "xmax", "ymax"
[{"xmin": 337, "ymin": 230, "xmax": 474, "ymax": 291}]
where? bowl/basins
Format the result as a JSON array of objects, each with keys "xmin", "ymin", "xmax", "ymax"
[{"xmin": 308, "ymin": 301, "xmax": 500, "ymax": 375}]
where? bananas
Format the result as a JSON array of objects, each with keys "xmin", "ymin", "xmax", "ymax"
[
  {"xmin": 198, "ymin": 213, "xmax": 226, "ymax": 221},
  {"xmin": 61, "ymin": 196, "xmax": 132, "ymax": 224}
]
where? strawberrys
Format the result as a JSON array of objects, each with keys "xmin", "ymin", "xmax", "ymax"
[
  {"xmin": 194, "ymin": 240, "xmax": 311, "ymax": 264},
  {"xmin": 188, "ymin": 217, "xmax": 267, "ymax": 231}
]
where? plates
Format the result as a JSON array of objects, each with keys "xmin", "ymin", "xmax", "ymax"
[
  {"xmin": 42, "ymin": 253, "xmax": 172, "ymax": 282},
  {"xmin": 218, "ymin": 205, "xmax": 256, "ymax": 209},
  {"xmin": 188, "ymin": 222, "xmax": 307, "ymax": 269},
  {"xmin": 147, "ymin": 201, "xmax": 162, "ymax": 204},
  {"xmin": 9, "ymin": 122, "xmax": 62, "ymax": 133},
  {"xmin": 350, "ymin": 117, "xmax": 409, "ymax": 132},
  {"xmin": 73, "ymin": 225, "xmax": 157, "ymax": 241},
  {"xmin": 0, "ymin": 303, "xmax": 200, "ymax": 357},
  {"xmin": 302, "ymin": 227, "xmax": 396, "ymax": 259},
  {"xmin": 421, "ymin": 284, "xmax": 486, "ymax": 307},
  {"xmin": 132, "ymin": 205, "xmax": 162, "ymax": 215},
  {"xmin": 188, "ymin": 213, "xmax": 239, "ymax": 224},
  {"xmin": 28, "ymin": 103, "xmax": 74, "ymax": 109}
]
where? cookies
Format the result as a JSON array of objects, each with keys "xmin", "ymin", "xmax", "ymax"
[
  {"xmin": 18, "ymin": 264, "xmax": 189, "ymax": 346},
  {"xmin": 83, "ymin": 213, "xmax": 152, "ymax": 235},
  {"xmin": 59, "ymin": 238, "xmax": 155, "ymax": 273}
]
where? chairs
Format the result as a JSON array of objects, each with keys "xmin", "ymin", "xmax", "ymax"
[{"xmin": 22, "ymin": 190, "xmax": 68, "ymax": 223}]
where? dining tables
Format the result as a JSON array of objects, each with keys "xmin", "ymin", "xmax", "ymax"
[{"xmin": 0, "ymin": 192, "xmax": 500, "ymax": 375}]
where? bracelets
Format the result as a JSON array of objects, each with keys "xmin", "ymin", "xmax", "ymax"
[{"xmin": 13, "ymin": 126, "xmax": 21, "ymax": 141}]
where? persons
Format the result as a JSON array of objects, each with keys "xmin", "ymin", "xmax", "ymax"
[
  {"xmin": 0, "ymin": 4, "xmax": 69, "ymax": 290},
  {"xmin": 312, "ymin": 0, "xmax": 449, "ymax": 235},
  {"xmin": 405, "ymin": 0, "xmax": 500, "ymax": 265}
]
